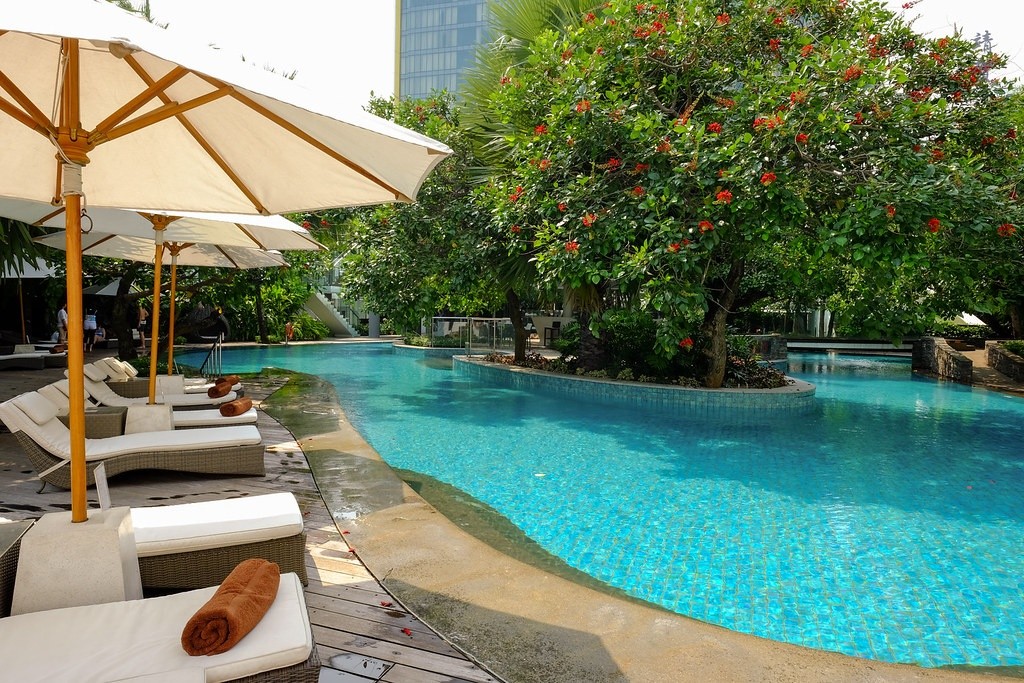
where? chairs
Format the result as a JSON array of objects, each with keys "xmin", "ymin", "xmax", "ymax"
[
  {"xmin": 93, "ymin": 357, "xmax": 242, "ymax": 394},
  {"xmin": 0, "ymin": 572, "xmax": 322, "ymax": 683},
  {"xmin": 63, "ymin": 363, "xmax": 237, "ymax": 411},
  {"xmin": 0, "ymin": 329, "xmax": 160, "ymax": 369},
  {"xmin": 39, "ymin": 377, "xmax": 259, "ymax": 429},
  {"xmin": 0, "ymin": 392, "xmax": 266, "ymax": 493},
  {"xmin": 0, "ymin": 493, "xmax": 309, "ymax": 585},
  {"xmin": 121, "ymin": 361, "xmax": 207, "ymax": 385}
]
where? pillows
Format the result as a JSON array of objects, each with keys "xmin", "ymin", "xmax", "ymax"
[
  {"xmin": 122, "ymin": 361, "xmax": 138, "ymax": 377},
  {"xmin": 54, "ymin": 379, "xmax": 90, "ymax": 400},
  {"xmin": 105, "ymin": 358, "xmax": 125, "ymax": 372},
  {"xmin": 12, "ymin": 391, "xmax": 58, "ymax": 425},
  {"xmin": 83, "ymin": 363, "xmax": 107, "ymax": 382}
]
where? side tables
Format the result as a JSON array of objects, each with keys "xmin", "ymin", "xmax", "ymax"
[
  {"xmin": 0, "ymin": 346, "xmax": 14, "ymax": 355},
  {"xmin": 66, "ymin": 406, "xmax": 128, "ymax": 439},
  {"xmin": 108, "ymin": 379, "xmax": 150, "ymax": 398},
  {"xmin": 0, "ymin": 519, "xmax": 35, "ymax": 618}
]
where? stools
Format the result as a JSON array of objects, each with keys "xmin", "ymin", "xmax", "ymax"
[{"xmin": 544, "ymin": 321, "xmax": 561, "ymax": 346}]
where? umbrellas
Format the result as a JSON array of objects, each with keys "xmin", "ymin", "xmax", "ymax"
[
  {"xmin": 0, "ymin": 196, "xmax": 333, "ymax": 403},
  {"xmin": 0, "ymin": 0, "xmax": 457, "ymax": 527},
  {"xmin": 31, "ymin": 229, "xmax": 292, "ymax": 377}
]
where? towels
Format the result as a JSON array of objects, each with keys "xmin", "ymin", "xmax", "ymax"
[
  {"xmin": 220, "ymin": 397, "xmax": 253, "ymax": 417},
  {"xmin": 215, "ymin": 376, "xmax": 240, "ymax": 386},
  {"xmin": 208, "ymin": 382, "xmax": 232, "ymax": 398},
  {"xmin": 180, "ymin": 556, "xmax": 281, "ymax": 657}
]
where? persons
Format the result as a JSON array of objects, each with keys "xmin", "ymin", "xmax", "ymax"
[
  {"xmin": 56, "ymin": 301, "xmax": 105, "ymax": 353},
  {"xmin": 138, "ymin": 303, "xmax": 148, "ymax": 349}
]
